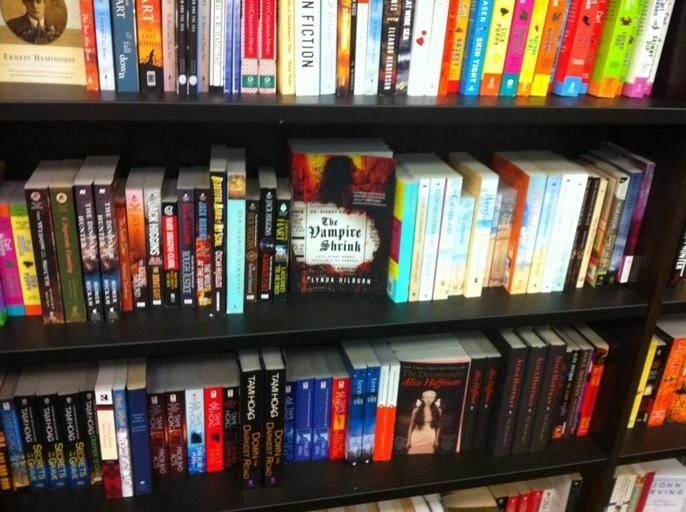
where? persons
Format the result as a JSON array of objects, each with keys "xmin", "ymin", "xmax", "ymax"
[
  {"xmin": 404, "ymin": 388, "xmax": 445, "ymax": 456},
  {"xmin": 5, "ymin": 0, "xmax": 64, "ymax": 46}
]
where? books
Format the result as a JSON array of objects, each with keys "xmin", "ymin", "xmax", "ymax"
[
  {"xmin": 0, "ymin": 310, "xmax": 686, "ymax": 512},
  {"xmin": 0, "ymin": 131, "xmax": 655, "ymax": 322},
  {"xmin": 0, "ymin": 0, "xmax": 674, "ymax": 102}
]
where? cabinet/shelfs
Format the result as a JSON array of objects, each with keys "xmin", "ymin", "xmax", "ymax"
[{"xmin": 2, "ymin": 0, "xmax": 686, "ymax": 512}]
